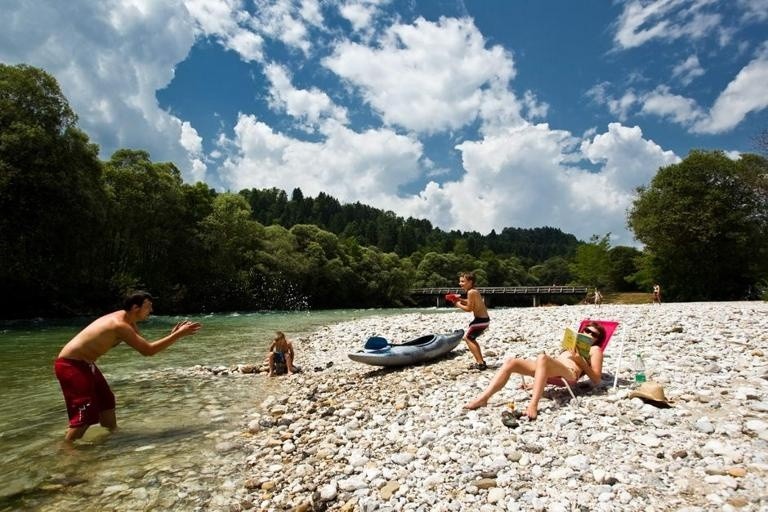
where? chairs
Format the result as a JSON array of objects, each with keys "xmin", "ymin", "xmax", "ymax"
[{"xmin": 522, "ymin": 320, "xmax": 625, "ymax": 403}]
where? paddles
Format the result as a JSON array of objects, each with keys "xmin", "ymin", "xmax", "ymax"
[{"xmin": 366, "ymin": 337, "xmax": 403, "ymax": 349}]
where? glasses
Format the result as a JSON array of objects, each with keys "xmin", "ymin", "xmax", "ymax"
[{"xmin": 584, "ymin": 327, "xmax": 599, "ymax": 338}]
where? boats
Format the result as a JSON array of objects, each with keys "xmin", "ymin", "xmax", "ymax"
[{"xmin": 346, "ymin": 329, "xmax": 464, "ymax": 367}]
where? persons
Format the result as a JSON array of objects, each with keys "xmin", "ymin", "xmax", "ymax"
[
  {"xmin": 451, "ymin": 272, "xmax": 490, "ymax": 371},
  {"xmin": 467, "ymin": 322, "xmax": 608, "ymax": 420},
  {"xmin": 55, "ymin": 292, "xmax": 204, "ymax": 458},
  {"xmin": 266, "ymin": 330, "xmax": 295, "ymax": 378},
  {"xmin": 593, "ymin": 286, "xmax": 603, "ymax": 308},
  {"xmin": 652, "ymin": 282, "xmax": 662, "ymax": 306}
]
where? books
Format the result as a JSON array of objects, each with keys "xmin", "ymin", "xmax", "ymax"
[{"xmin": 560, "ymin": 327, "xmax": 593, "ymax": 360}]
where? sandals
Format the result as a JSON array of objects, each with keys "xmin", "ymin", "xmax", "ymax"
[
  {"xmin": 502, "ymin": 409, "xmax": 522, "ymax": 427},
  {"xmin": 467, "ymin": 361, "xmax": 487, "ymax": 370}
]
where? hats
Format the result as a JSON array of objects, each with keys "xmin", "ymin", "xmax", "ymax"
[{"xmin": 627, "ymin": 381, "xmax": 676, "ymax": 408}]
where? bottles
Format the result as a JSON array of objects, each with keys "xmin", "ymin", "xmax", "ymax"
[{"xmin": 634, "ymin": 354, "xmax": 646, "ymax": 385}]
